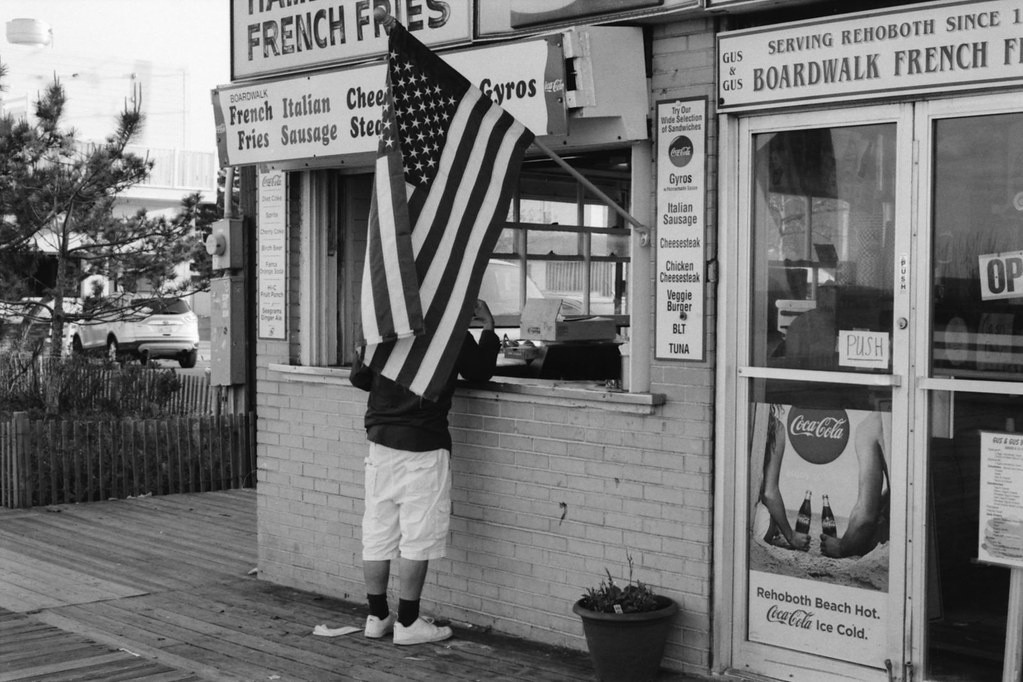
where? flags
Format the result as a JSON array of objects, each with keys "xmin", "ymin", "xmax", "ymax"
[{"xmin": 351, "ymin": 26, "xmax": 539, "ymax": 418}]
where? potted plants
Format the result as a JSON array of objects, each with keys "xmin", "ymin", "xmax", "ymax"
[{"xmin": 573, "ymin": 556, "xmax": 679, "ymax": 682}]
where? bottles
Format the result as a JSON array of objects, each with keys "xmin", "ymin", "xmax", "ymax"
[
  {"xmin": 795, "ymin": 490, "xmax": 813, "ymax": 534},
  {"xmin": 822, "ymin": 495, "xmax": 837, "ymax": 538}
]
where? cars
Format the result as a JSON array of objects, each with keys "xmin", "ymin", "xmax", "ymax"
[
  {"xmin": 72, "ymin": 291, "xmax": 198, "ymax": 368},
  {"xmin": 60, "ymin": 313, "xmax": 62, "ymax": 316},
  {"xmin": 0, "ymin": 298, "xmax": 84, "ymax": 346},
  {"xmin": 467, "ymin": 259, "xmax": 545, "ymax": 369}
]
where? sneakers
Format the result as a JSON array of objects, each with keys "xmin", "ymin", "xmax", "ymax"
[
  {"xmin": 393, "ymin": 613, "xmax": 453, "ymax": 645},
  {"xmin": 364, "ymin": 610, "xmax": 398, "ymax": 638}
]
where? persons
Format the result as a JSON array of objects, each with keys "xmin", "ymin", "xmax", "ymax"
[
  {"xmin": 348, "ymin": 293, "xmax": 501, "ymax": 646},
  {"xmin": 761, "ymin": 404, "xmax": 900, "ymax": 559}
]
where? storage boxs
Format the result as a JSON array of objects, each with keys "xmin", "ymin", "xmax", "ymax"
[
  {"xmin": 504, "ymin": 345, "xmax": 543, "ymax": 360},
  {"xmin": 520, "ymin": 297, "xmax": 617, "ymax": 341}
]
